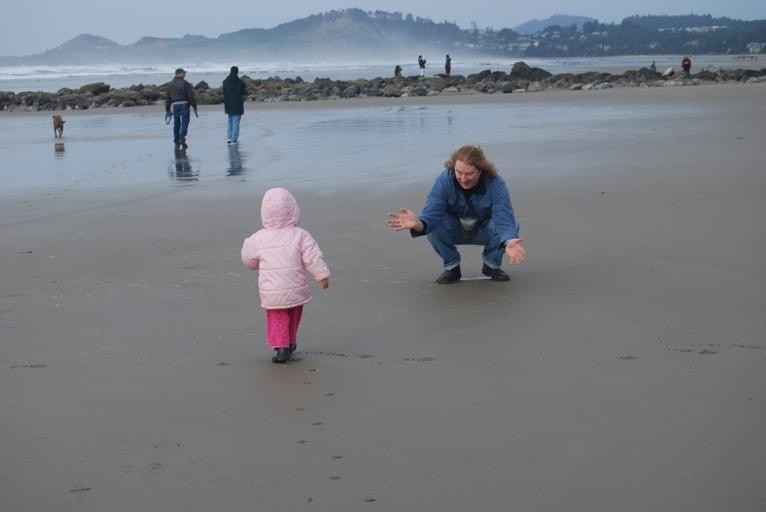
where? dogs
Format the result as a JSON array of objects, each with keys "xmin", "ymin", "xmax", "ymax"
[{"xmin": 52, "ymin": 115, "xmax": 67, "ymax": 138}]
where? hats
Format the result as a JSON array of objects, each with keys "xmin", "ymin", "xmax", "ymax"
[{"xmin": 173, "ymin": 68, "xmax": 187, "ymax": 79}]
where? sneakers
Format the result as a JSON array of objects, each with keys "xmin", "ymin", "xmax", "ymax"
[{"xmin": 271, "ymin": 343, "xmax": 297, "ymax": 363}]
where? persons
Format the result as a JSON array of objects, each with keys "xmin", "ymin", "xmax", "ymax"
[
  {"xmin": 395, "ymin": 65, "xmax": 402, "ymax": 75},
  {"xmin": 225, "ymin": 141, "xmax": 248, "ymax": 181},
  {"xmin": 221, "ymin": 65, "xmax": 248, "ymax": 145},
  {"xmin": 418, "ymin": 55, "xmax": 426, "ymax": 76},
  {"xmin": 445, "ymin": 53, "xmax": 452, "ymax": 75},
  {"xmin": 681, "ymin": 54, "xmax": 691, "ymax": 74},
  {"xmin": 385, "ymin": 143, "xmax": 526, "ymax": 284},
  {"xmin": 165, "ymin": 68, "xmax": 199, "ymax": 149},
  {"xmin": 649, "ymin": 61, "xmax": 656, "ymax": 69},
  {"xmin": 166, "ymin": 143, "xmax": 200, "ymax": 184},
  {"xmin": 241, "ymin": 185, "xmax": 335, "ymax": 365}
]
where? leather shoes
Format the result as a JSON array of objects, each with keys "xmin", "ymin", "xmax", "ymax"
[
  {"xmin": 481, "ymin": 262, "xmax": 510, "ymax": 281},
  {"xmin": 436, "ymin": 264, "xmax": 462, "ymax": 284}
]
